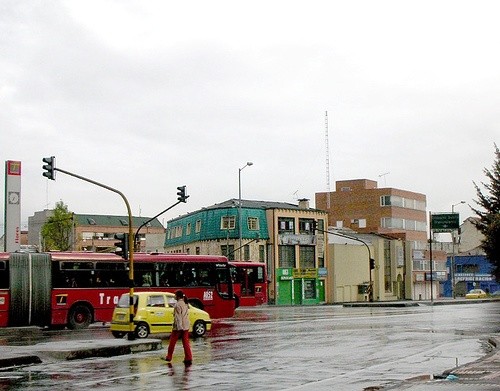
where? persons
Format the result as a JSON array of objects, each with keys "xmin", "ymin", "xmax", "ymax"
[
  {"xmin": 160, "ymin": 290, "xmax": 193, "ymax": 363},
  {"xmin": 60, "ymin": 262, "xmax": 259, "ymax": 297}
]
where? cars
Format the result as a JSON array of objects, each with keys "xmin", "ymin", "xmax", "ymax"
[
  {"xmin": 465, "ymin": 288, "xmax": 488, "ymax": 299},
  {"xmin": 109, "ymin": 291, "xmax": 212, "ymax": 339}
]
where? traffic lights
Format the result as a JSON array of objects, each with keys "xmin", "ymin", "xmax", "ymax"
[
  {"xmin": 313, "ymin": 221, "xmax": 318, "ymax": 228},
  {"xmin": 370, "ymin": 259, "xmax": 376, "ymax": 269},
  {"xmin": 42, "ymin": 156, "xmax": 53, "ymax": 179},
  {"xmin": 114, "ymin": 234, "xmax": 128, "ymax": 259}
]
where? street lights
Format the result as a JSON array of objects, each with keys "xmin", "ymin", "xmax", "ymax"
[
  {"xmin": 238, "ymin": 162, "xmax": 254, "ymax": 261},
  {"xmin": 176, "ymin": 186, "xmax": 186, "ymax": 202},
  {"xmin": 451, "ymin": 200, "xmax": 466, "ymax": 299}
]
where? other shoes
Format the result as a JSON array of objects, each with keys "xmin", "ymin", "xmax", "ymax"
[
  {"xmin": 181, "ymin": 360, "xmax": 193, "ymax": 364},
  {"xmin": 159, "ymin": 356, "xmax": 172, "ymax": 362}
]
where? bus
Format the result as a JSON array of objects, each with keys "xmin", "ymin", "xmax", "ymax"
[
  {"xmin": 216, "ymin": 260, "xmax": 272, "ymax": 310},
  {"xmin": 0, "ymin": 249, "xmax": 235, "ymax": 330}
]
